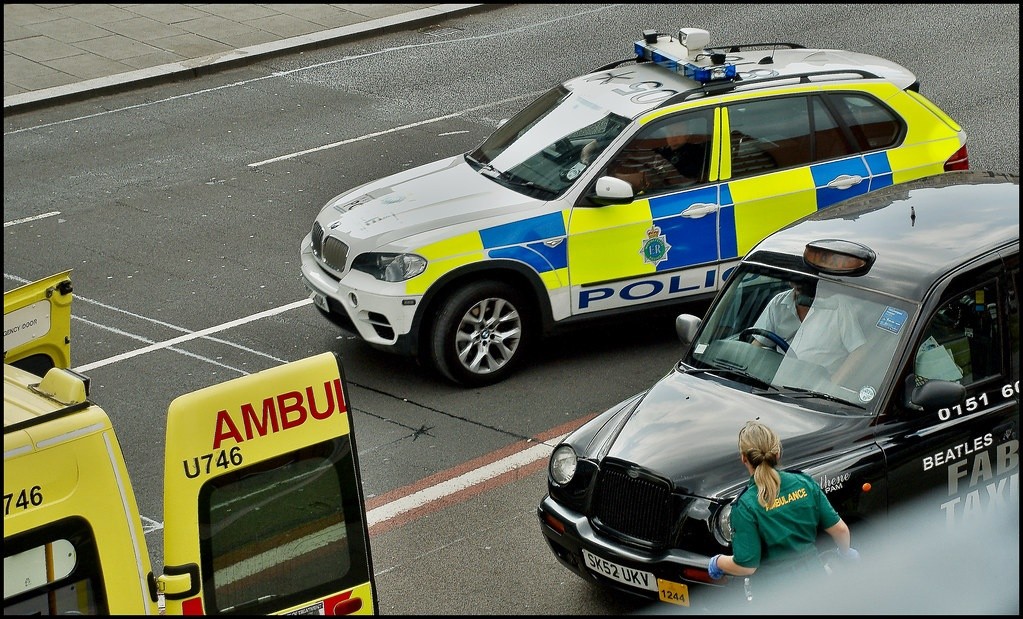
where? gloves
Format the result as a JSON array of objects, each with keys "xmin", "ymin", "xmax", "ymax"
[{"xmin": 708, "ymin": 554, "xmax": 725, "ymax": 580}]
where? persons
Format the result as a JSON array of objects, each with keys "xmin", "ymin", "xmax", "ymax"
[
  {"xmin": 644, "ymin": 120, "xmax": 706, "ymax": 193},
  {"xmin": 709, "ymin": 421, "xmax": 859, "ymax": 602},
  {"xmin": 580, "ymin": 123, "xmax": 644, "ymax": 167},
  {"xmin": 750, "ymin": 278, "xmax": 869, "ymax": 385}
]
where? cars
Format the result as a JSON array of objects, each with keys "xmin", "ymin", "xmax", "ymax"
[{"xmin": 300, "ymin": 25, "xmax": 969, "ymax": 390}]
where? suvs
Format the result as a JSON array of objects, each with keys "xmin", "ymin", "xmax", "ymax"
[{"xmin": 534, "ymin": 167, "xmax": 1023, "ymax": 608}]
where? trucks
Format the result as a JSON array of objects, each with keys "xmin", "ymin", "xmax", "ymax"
[{"xmin": 3, "ymin": 265, "xmax": 393, "ymax": 619}]
